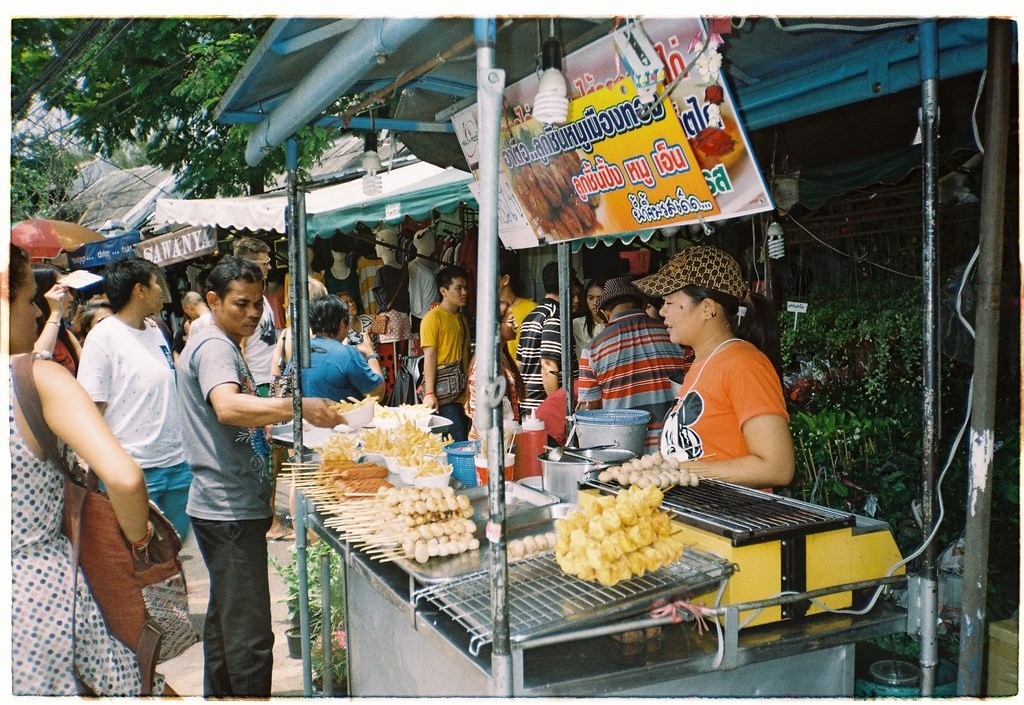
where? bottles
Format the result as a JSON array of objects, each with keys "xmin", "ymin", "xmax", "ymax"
[{"xmin": 504, "ymin": 409, "xmax": 548, "ymax": 485}]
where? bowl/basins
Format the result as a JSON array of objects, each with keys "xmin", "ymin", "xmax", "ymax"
[
  {"xmin": 869, "ymin": 660, "xmax": 920, "ymax": 688},
  {"xmin": 340, "ymin": 403, "xmax": 453, "ymax": 486}
]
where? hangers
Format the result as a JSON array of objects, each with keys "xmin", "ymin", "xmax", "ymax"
[{"xmin": 437, "ymin": 220, "xmax": 473, "ymax": 244}]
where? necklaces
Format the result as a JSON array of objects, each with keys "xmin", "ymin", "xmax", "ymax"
[{"xmin": 511, "ymin": 296, "xmax": 517, "ymax": 305}]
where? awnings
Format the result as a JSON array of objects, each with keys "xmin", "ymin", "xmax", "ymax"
[
  {"xmin": 153, "ymin": 162, "xmax": 478, "ymax": 244},
  {"xmin": 67, "ymin": 226, "xmax": 218, "ymax": 267}
]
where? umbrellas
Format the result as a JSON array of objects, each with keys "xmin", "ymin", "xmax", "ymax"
[{"xmin": 11, "ymin": 219, "xmax": 106, "ymax": 260}]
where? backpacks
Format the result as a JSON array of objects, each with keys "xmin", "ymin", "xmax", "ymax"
[{"xmin": 11, "ymin": 350, "xmax": 199, "ymax": 696}]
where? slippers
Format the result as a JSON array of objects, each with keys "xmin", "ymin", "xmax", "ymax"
[{"xmin": 267, "ymin": 528, "xmax": 295, "ymax": 542}]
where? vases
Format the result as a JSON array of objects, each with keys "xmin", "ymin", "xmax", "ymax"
[{"xmin": 313, "ymin": 678, "xmax": 347, "ymax": 694}]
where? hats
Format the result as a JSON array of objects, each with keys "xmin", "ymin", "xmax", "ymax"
[
  {"xmin": 631, "ymin": 244, "xmax": 746, "ymax": 300},
  {"xmin": 596, "ymin": 276, "xmax": 648, "ymax": 323}
]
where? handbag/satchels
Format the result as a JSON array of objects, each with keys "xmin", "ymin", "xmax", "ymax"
[
  {"xmin": 371, "ymin": 314, "xmax": 388, "ymax": 334},
  {"xmin": 416, "ymin": 358, "xmax": 468, "ymax": 407},
  {"xmin": 270, "ymin": 342, "xmax": 315, "ymax": 426}
]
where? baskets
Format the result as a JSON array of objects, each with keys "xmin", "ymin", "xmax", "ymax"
[
  {"xmin": 443, "ymin": 441, "xmax": 482, "ymax": 488},
  {"xmin": 574, "ymin": 409, "xmax": 650, "ymax": 424}
]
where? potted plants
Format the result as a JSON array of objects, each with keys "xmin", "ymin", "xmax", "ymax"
[{"xmin": 268, "ymin": 546, "xmax": 315, "ymax": 659}]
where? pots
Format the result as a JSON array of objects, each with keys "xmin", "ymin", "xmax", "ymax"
[{"xmin": 537, "ymin": 449, "xmax": 636, "ymax": 509}]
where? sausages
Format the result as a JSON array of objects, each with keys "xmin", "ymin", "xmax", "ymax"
[{"xmin": 319, "ymin": 458, "xmax": 395, "ymax": 503}]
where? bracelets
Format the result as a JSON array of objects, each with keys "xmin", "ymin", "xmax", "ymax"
[
  {"xmin": 424, "ymin": 392, "xmax": 434, "ymax": 396},
  {"xmin": 46, "ymin": 320, "xmax": 61, "ymax": 327},
  {"xmin": 133, "ymin": 522, "xmax": 154, "ymax": 551},
  {"xmin": 367, "ymin": 354, "xmax": 381, "ymax": 362}
]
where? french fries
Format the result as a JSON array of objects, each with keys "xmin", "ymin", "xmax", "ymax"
[{"xmin": 325, "ymin": 393, "xmax": 455, "ymax": 479}]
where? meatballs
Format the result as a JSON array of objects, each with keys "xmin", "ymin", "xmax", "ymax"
[
  {"xmin": 599, "ymin": 451, "xmax": 699, "ymax": 491},
  {"xmin": 368, "ymin": 486, "xmax": 481, "ymax": 563},
  {"xmin": 508, "ymin": 532, "xmax": 557, "ymax": 557}
]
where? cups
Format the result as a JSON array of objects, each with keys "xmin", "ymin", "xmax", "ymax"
[{"xmin": 475, "ymin": 452, "xmax": 515, "ymax": 484}]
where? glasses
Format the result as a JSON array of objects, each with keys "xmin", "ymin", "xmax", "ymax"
[{"xmin": 251, "ymin": 257, "xmax": 271, "ymax": 267}]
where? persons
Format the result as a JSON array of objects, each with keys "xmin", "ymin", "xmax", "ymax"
[
  {"xmin": 11, "ymin": 241, "xmax": 179, "ymax": 697},
  {"xmin": 420, "ymin": 266, "xmax": 474, "ymax": 441},
  {"xmin": 264, "ymin": 227, "xmax": 439, "ymax": 333},
  {"xmin": 629, "ymin": 246, "xmax": 795, "ymax": 493},
  {"xmin": 174, "ymin": 254, "xmax": 349, "ymax": 699},
  {"xmin": 462, "ymin": 261, "xmax": 695, "ymax": 458},
  {"xmin": 30, "ymin": 237, "xmax": 385, "ymax": 539}
]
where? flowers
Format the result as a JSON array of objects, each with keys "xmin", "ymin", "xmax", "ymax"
[
  {"xmin": 311, "ymin": 631, "xmax": 346, "ymax": 686},
  {"xmin": 775, "ymin": 281, "xmax": 951, "ymax": 458}
]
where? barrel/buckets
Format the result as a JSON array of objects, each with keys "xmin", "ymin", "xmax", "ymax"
[{"xmin": 574, "ymin": 422, "xmax": 647, "ymax": 459}]
